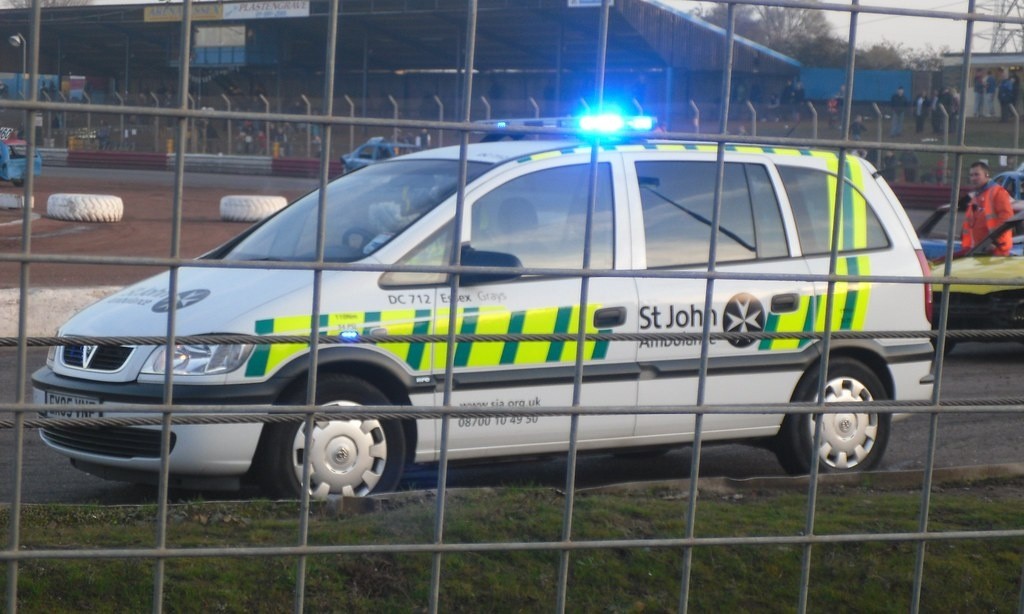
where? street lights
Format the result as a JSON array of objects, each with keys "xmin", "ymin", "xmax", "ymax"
[{"xmin": 10, "ymin": 32, "xmax": 28, "ymax": 140}]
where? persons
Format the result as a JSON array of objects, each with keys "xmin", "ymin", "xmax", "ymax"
[
  {"xmin": 848, "ymin": 134, "xmax": 918, "ymax": 185},
  {"xmin": 962, "ymin": 161, "xmax": 1014, "ymax": 257},
  {"xmin": 851, "ymin": 115, "xmax": 867, "ymax": 141},
  {"xmin": 1, "ymin": 81, "xmax": 322, "ymax": 158},
  {"xmin": 913, "ymin": 86, "xmax": 962, "ymax": 135},
  {"xmin": 973, "ymin": 65, "xmax": 1018, "ymax": 122},
  {"xmin": 748, "ymin": 76, "xmax": 804, "ymax": 123},
  {"xmin": 827, "ymin": 84, "xmax": 846, "ymax": 129},
  {"xmin": 390, "ymin": 127, "xmax": 431, "ymax": 154},
  {"xmin": 888, "ymin": 86, "xmax": 907, "ymax": 138}
]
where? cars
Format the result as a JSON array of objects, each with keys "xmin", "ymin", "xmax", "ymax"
[
  {"xmin": 991, "ymin": 165, "xmax": 1024, "ymax": 200},
  {"xmin": 927, "ymin": 212, "xmax": 1024, "ymax": 354},
  {"xmin": 340, "ymin": 142, "xmax": 419, "ymax": 174},
  {"xmin": 915, "ymin": 202, "xmax": 1024, "ymax": 261}
]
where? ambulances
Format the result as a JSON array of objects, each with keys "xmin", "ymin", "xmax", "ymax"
[{"xmin": 31, "ymin": 115, "xmax": 935, "ymax": 498}]
